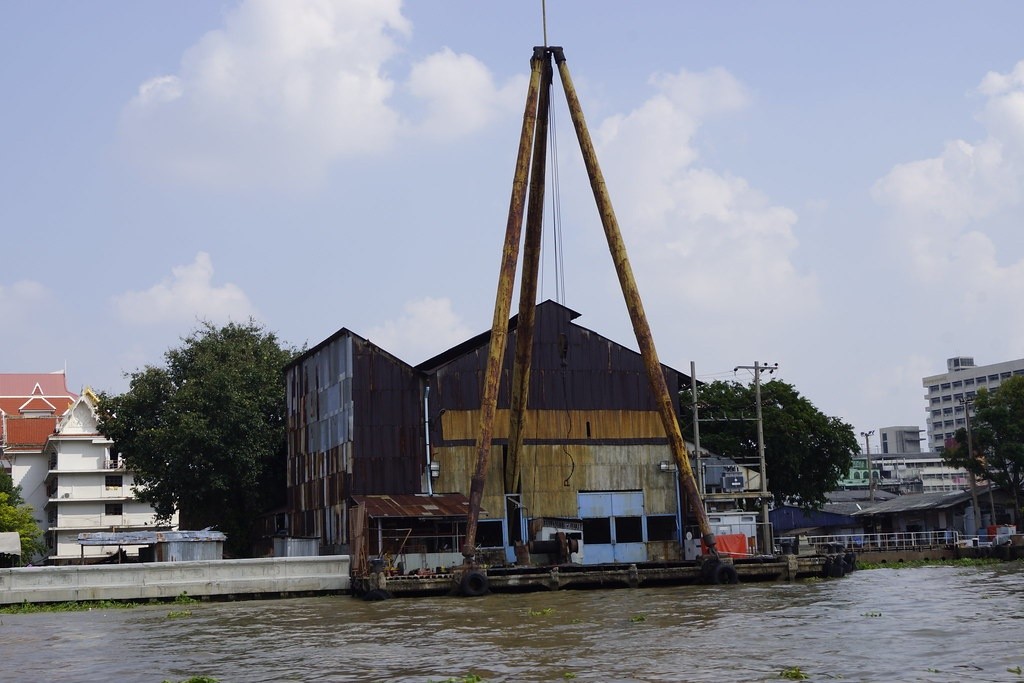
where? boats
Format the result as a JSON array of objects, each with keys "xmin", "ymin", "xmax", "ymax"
[{"xmin": 350, "ymin": 0, "xmax": 863, "ymax": 599}]
